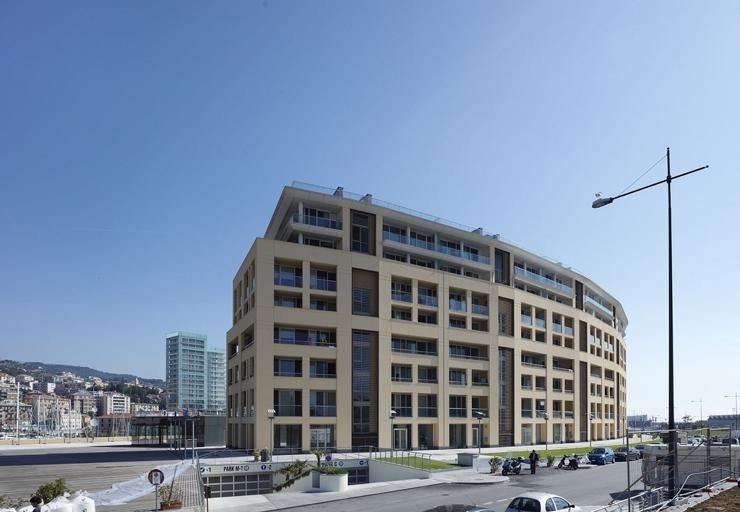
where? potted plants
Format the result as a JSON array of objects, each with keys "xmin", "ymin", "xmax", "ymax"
[
  {"xmin": 488, "ymin": 457, "xmax": 503, "ymax": 473},
  {"xmin": 151, "ymin": 483, "xmax": 184, "ymax": 510},
  {"xmin": 319, "ymin": 464, "xmax": 349, "ymax": 492},
  {"xmin": 546, "ymin": 452, "xmax": 557, "ymax": 467}
]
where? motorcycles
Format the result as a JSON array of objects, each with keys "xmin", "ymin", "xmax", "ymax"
[
  {"xmin": 502, "ymin": 457, "xmax": 524, "ymax": 475},
  {"xmin": 557, "ymin": 455, "xmax": 579, "ymax": 470}
]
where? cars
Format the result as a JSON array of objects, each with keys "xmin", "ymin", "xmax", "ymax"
[
  {"xmin": 505, "ymin": 490, "xmax": 584, "ymax": 512},
  {"xmin": 589, "ymin": 444, "xmax": 645, "ymax": 465},
  {"xmin": 687, "ymin": 435, "xmax": 739, "ymax": 446}
]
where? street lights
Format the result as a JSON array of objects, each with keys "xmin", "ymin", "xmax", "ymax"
[
  {"xmin": 266, "ymin": 408, "xmax": 277, "ymax": 461},
  {"xmin": 724, "ymin": 392, "xmax": 739, "ymax": 430},
  {"xmin": 475, "ymin": 410, "xmax": 486, "ymax": 455},
  {"xmin": 593, "ymin": 146, "xmax": 708, "ymax": 498},
  {"xmin": 690, "ymin": 398, "xmax": 704, "ymax": 435},
  {"xmin": 543, "ymin": 411, "xmax": 549, "ymax": 450},
  {"xmin": 388, "ymin": 410, "xmax": 397, "ymax": 459}
]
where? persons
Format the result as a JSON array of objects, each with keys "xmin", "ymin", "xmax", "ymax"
[{"xmin": 529, "ymin": 449, "xmax": 539, "ymax": 474}]
